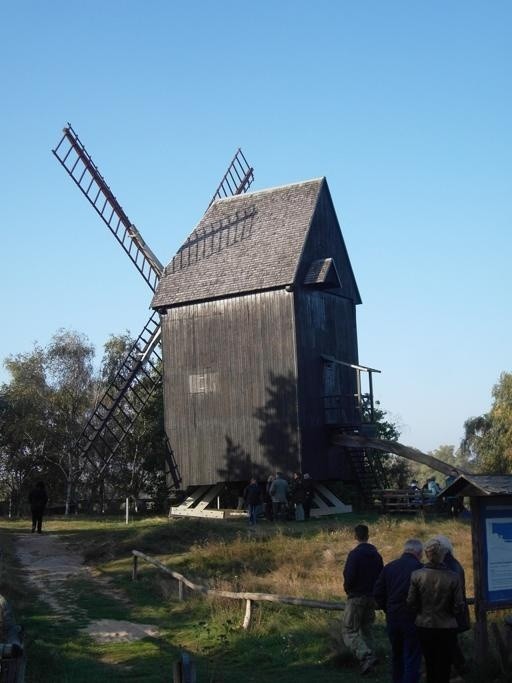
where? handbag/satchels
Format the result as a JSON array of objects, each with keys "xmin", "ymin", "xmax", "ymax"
[{"xmin": 351, "ymin": 602, "xmax": 375, "ymax": 621}]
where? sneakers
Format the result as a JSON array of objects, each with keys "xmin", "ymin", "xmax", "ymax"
[{"xmin": 361, "ymin": 656, "xmax": 379, "ymax": 676}]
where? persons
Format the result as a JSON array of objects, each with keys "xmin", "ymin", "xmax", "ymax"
[
  {"xmin": 242, "ymin": 470, "xmax": 316, "ymax": 524},
  {"xmin": 408, "ymin": 476, "xmax": 443, "ymax": 507},
  {"xmin": 401, "ymin": 538, "xmax": 468, "ymax": 682},
  {"xmin": 28, "ymin": 480, "xmax": 49, "ymax": 534},
  {"xmin": 339, "ymin": 523, "xmax": 386, "ymax": 677},
  {"xmin": 431, "ymin": 532, "xmax": 472, "ymax": 682},
  {"xmin": 371, "ymin": 537, "xmax": 425, "ymax": 682}
]
